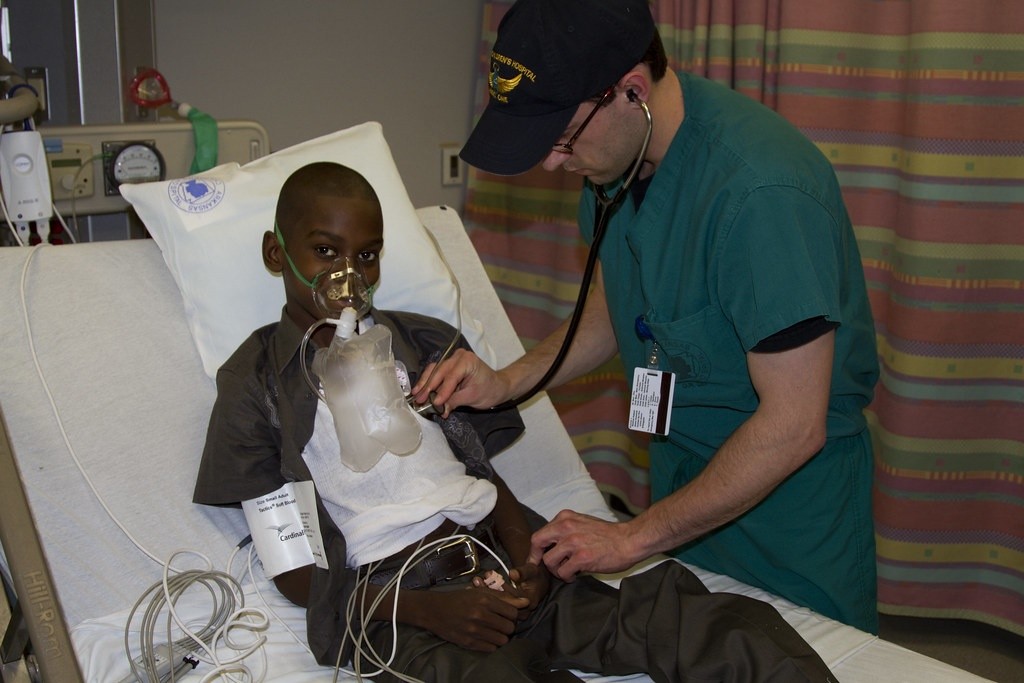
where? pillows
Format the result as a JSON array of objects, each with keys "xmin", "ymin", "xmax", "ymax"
[{"xmin": 116, "ymin": 121, "xmax": 497, "ymax": 390}]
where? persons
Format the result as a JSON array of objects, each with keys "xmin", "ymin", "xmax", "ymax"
[
  {"xmin": 193, "ymin": 160, "xmax": 838, "ymax": 682},
  {"xmin": 410, "ymin": 1, "xmax": 881, "ymax": 632}
]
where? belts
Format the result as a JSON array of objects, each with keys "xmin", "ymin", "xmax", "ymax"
[{"xmin": 396, "ymin": 522, "xmax": 500, "ymax": 592}]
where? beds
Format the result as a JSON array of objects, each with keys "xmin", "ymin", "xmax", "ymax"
[{"xmin": 0, "ymin": 205, "xmax": 996, "ymax": 683}]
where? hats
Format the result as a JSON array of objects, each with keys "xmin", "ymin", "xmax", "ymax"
[{"xmin": 460, "ymin": 0, "xmax": 657, "ymax": 176}]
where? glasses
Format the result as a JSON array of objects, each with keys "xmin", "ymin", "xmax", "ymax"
[{"xmin": 552, "ymin": 83, "xmax": 618, "ymax": 153}]
